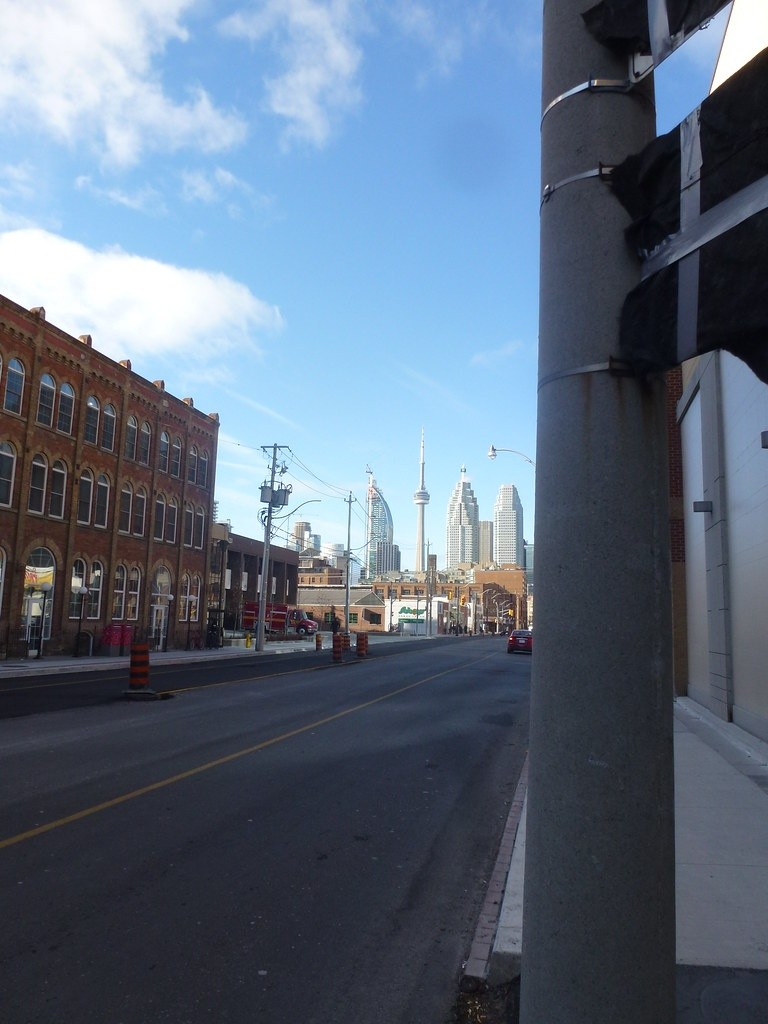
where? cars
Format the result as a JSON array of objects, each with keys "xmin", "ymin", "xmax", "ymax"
[{"xmin": 507, "ymin": 628, "xmax": 532, "ymax": 654}]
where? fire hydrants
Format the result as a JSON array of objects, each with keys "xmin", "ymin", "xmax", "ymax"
[{"xmin": 245, "ymin": 634, "xmax": 252, "ymax": 648}]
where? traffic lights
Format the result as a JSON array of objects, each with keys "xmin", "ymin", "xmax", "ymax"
[{"xmin": 461, "ymin": 596, "xmax": 464, "ymax": 605}]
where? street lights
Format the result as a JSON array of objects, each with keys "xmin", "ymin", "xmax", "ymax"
[
  {"xmin": 184, "ymin": 594, "xmax": 197, "ymax": 652},
  {"xmin": 71, "ymin": 586, "xmax": 88, "ymax": 657},
  {"xmin": 480, "ymin": 588, "xmax": 514, "ymax": 637},
  {"xmin": 36, "ymin": 582, "xmax": 52, "ymax": 660},
  {"xmin": 343, "ymin": 536, "xmax": 379, "ymax": 645},
  {"xmin": 255, "ymin": 499, "xmax": 323, "ymax": 652},
  {"xmin": 161, "ymin": 593, "xmax": 175, "ymax": 652},
  {"xmin": 455, "ymin": 580, "xmax": 474, "ymax": 637}
]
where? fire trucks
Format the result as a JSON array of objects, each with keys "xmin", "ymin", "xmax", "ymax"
[{"xmin": 240, "ymin": 600, "xmax": 319, "ymax": 637}]
